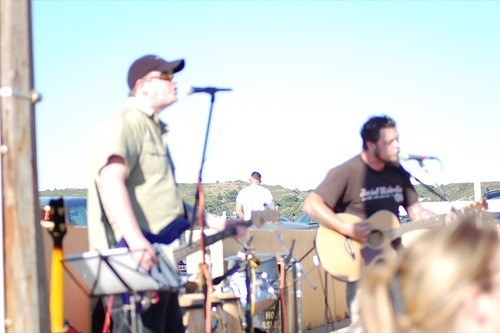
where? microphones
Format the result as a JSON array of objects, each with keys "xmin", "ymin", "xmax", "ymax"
[
  {"xmin": 402, "ymin": 155, "xmax": 438, "ymax": 162},
  {"xmin": 182, "ymin": 85, "xmax": 233, "ymax": 95}
]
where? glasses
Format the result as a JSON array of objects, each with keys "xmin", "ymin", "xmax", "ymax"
[{"xmin": 145, "ymin": 71, "xmax": 173, "ymax": 80}]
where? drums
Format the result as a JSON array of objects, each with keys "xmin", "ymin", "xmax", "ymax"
[
  {"xmin": 222, "ymin": 267, "xmax": 276, "ymax": 320},
  {"xmin": 177, "ymin": 294, "xmax": 248, "ymax": 333},
  {"xmin": 227, "ymin": 255, "xmax": 281, "ymax": 301}
]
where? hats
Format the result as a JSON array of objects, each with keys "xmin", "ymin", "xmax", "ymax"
[{"xmin": 128, "ymin": 54, "xmax": 185, "ymax": 88}]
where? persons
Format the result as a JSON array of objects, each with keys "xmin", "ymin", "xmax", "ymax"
[
  {"xmin": 300, "ymin": 115, "xmax": 468, "ymax": 333},
  {"xmin": 234, "ymin": 171, "xmax": 275, "ymax": 224},
  {"xmin": 353, "ymin": 204, "xmax": 500, "ymax": 333},
  {"xmin": 87, "ymin": 54, "xmax": 187, "ymax": 333}
]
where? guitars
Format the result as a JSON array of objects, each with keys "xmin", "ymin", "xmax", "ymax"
[
  {"xmin": 113, "ymin": 217, "xmax": 253, "ymax": 313},
  {"xmin": 314, "ymin": 197, "xmax": 490, "ymax": 282},
  {"xmin": 43, "ymin": 197, "xmax": 68, "ymax": 333}
]
